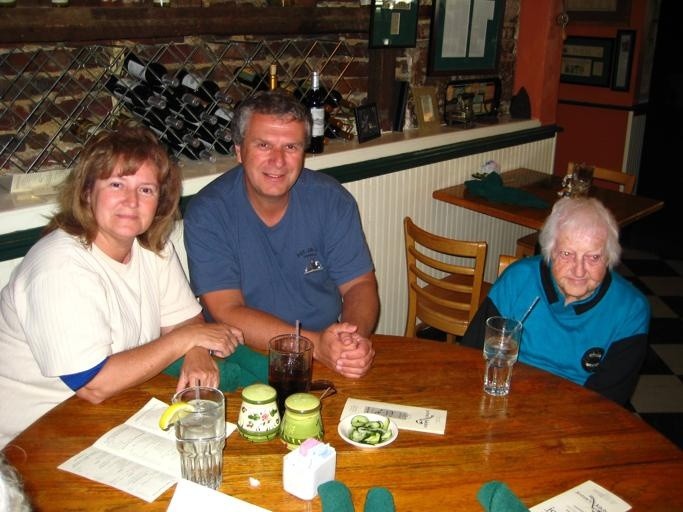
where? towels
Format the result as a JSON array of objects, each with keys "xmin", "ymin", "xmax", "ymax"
[{"xmin": 317, "ymin": 476, "xmax": 531, "ymax": 512}]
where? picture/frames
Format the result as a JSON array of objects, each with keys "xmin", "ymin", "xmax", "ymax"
[
  {"xmin": 560, "ymin": 35, "xmax": 618, "ymax": 88},
  {"xmin": 565, "ymin": 0, "xmax": 631, "ymax": 26},
  {"xmin": 368, "ymin": 0, "xmax": 419, "ymax": 49},
  {"xmin": 354, "ymin": 102, "xmax": 381, "ymax": 144},
  {"xmin": 426, "ymin": 0, "xmax": 506, "ymax": 77},
  {"xmin": 412, "ymin": 87, "xmax": 441, "ymax": 131},
  {"xmin": 610, "ymin": 29, "xmax": 637, "ymax": 92}
]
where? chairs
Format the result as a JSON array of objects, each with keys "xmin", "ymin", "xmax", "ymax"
[
  {"xmin": 404, "ymin": 216, "xmax": 492, "ymax": 344},
  {"xmin": 516, "ymin": 163, "xmax": 636, "ymax": 257},
  {"xmin": 497, "ymin": 255, "xmax": 521, "ymax": 279}
]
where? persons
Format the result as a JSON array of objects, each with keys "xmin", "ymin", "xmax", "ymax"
[
  {"xmin": 184, "ymin": 93, "xmax": 380, "ymax": 379},
  {"xmin": 0, "ymin": 124, "xmax": 244, "ymax": 448},
  {"xmin": 461, "ymin": 196, "xmax": 651, "ymax": 407}
]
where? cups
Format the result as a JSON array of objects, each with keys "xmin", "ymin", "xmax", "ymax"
[
  {"xmin": 280, "ymin": 392, "xmax": 324, "ymax": 451},
  {"xmin": 237, "ymin": 385, "xmax": 280, "ymax": 442},
  {"xmin": 267, "ymin": 334, "xmax": 314, "ymax": 415},
  {"xmin": 571, "ymin": 163, "xmax": 594, "ymax": 201},
  {"xmin": 482, "ymin": 317, "xmax": 524, "ymax": 394},
  {"xmin": 168, "ymin": 383, "xmax": 225, "ymax": 490}
]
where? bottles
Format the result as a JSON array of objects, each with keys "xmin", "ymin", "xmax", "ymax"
[
  {"xmin": 185, "ymin": 103, "xmax": 216, "ymax": 125},
  {"xmin": 149, "ymin": 125, "xmax": 200, "ymax": 149},
  {"xmin": 319, "ymin": 86, "xmax": 356, "ymax": 111},
  {"xmin": 125, "ymin": 57, "xmax": 178, "ymax": 87},
  {"xmin": 236, "ymin": 65, "xmax": 294, "ymax": 96},
  {"xmin": 188, "ymin": 121, "xmax": 230, "ymax": 143},
  {"xmin": 170, "ymin": 141, "xmax": 216, "ymax": 162},
  {"xmin": 150, "ymin": 107, "xmax": 182, "ymax": 130},
  {"xmin": 277, "ymin": 78, "xmax": 304, "ymax": 104},
  {"xmin": 325, "ymin": 124, "xmax": 353, "ymax": 141},
  {"xmin": 267, "ymin": 63, "xmax": 279, "ymax": 91},
  {"xmin": 169, "ymin": 88, "xmax": 200, "ymax": 107},
  {"xmin": 176, "ymin": 69, "xmax": 232, "ymax": 106},
  {"xmin": 325, "ymin": 116, "xmax": 342, "ymax": 130},
  {"xmin": 319, "ymin": 101, "xmax": 333, "ymax": 113},
  {"xmin": 111, "ymin": 77, "xmax": 164, "ymax": 109},
  {"xmin": 302, "ymin": 70, "xmax": 327, "ymax": 152}
]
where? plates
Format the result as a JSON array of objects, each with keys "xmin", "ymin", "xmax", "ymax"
[{"xmin": 336, "ymin": 413, "xmax": 398, "ymax": 451}]
